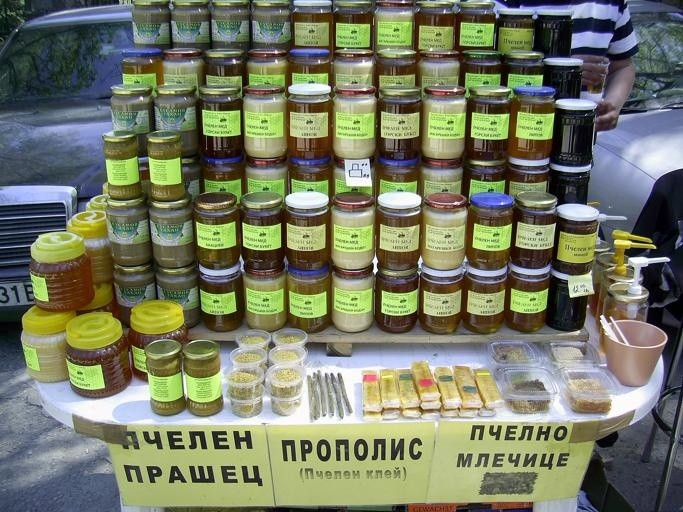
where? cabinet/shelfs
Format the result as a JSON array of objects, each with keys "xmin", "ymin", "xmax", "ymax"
[{"xmin": 35, "ymin": 321, "xmax": 667, "ymax": 512}]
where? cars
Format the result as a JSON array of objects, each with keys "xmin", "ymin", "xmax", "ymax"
[
  {"xmin": 579, "ymin": 0, "xmax": 682, "ymax": 320},
  {"xmin": 0, "ymin": 0, "xmax": 151, "ymax": 332}
]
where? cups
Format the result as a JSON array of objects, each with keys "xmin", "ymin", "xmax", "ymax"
[
  {"xmin": 604, "ymin": 321, "xmax": 668, "ymax": 388},
  {"xmin": 225, "ymin": 329, "xmax": 307, "ymax": 417},
  {"xmin": 585, "ymin": 54, "xmax": 610, "ymax": 95}
]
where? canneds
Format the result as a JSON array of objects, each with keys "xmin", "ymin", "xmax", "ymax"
[
  {"xmin": 128, "ymin": 299, "xmax": 189, "ymax": 381},
  {"xmin": 144, "ymin": 339, "xmax": 186, "ymax": 416},
  {"xmin": 66, "ymin": 0, "xmax": 601, "ymax": 336},
  {"xmin": 65, "ymin": 311, "xmax": 132, "ymax": 398},
  {"xmin": 22, "ymin": 305, "xmax": 77, "ymax": 383},
  {"xmin": 29, "ymin": 232, "xmax": 95, "ymax": 313},
  {"xmin": 226, "ymin": 329, "xmax": 309, "ymax": 418},
  {"xmin": 182, "ymin": 339, "xmax": 224, "ymax": 416}
]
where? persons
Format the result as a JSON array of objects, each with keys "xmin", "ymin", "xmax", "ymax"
[{"xmin": 494, "ymin": 0, "xmax": 640, "ymax": 130}]
